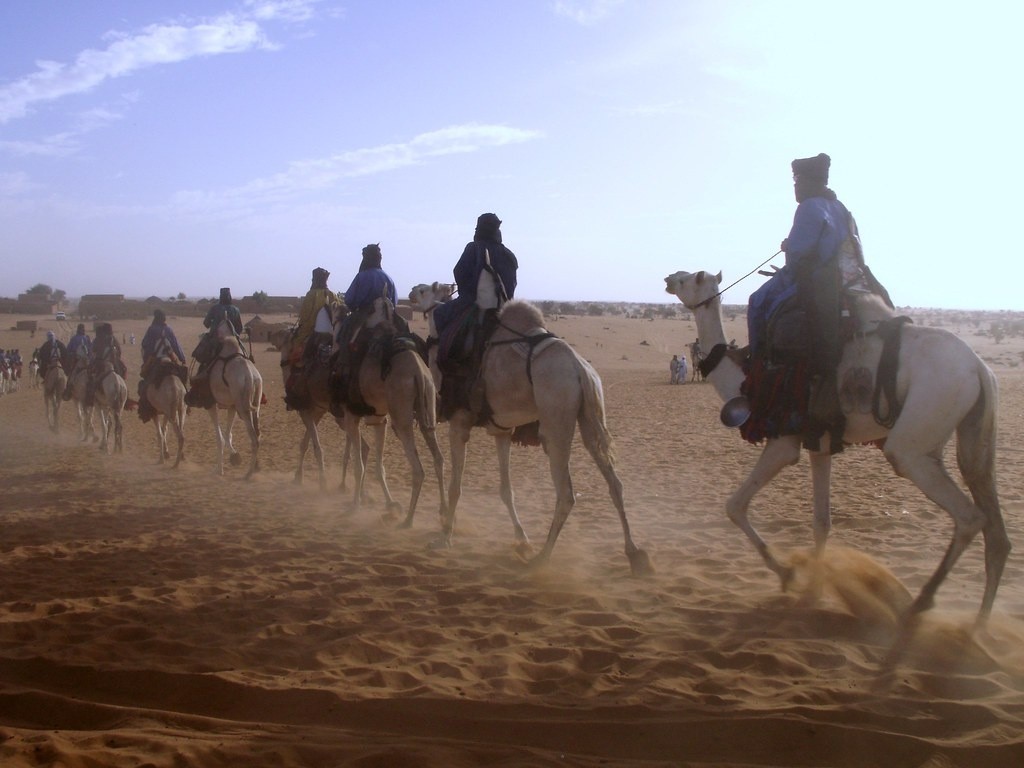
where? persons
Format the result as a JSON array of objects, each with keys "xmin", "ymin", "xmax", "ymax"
[
  {"xmin": 747, "ymin": 154, "xmax": 861, "ymax": 359},
  {"xmin": 38, "ymin": 331, "xmax": 67, "ymax": 378},
  {"xmin": 676, "ymin": 356, "xmax": 688, "ymax": 384},
  {"xmin": 0, "ymin": 348, "xmax": 22, "ymax": 369},
  {"xmin": 433, "ymin": 213, "xmax": 518, "ymax": 338},
  {"xmin": 90, "ymin": 323, "xmax": 128, "ymax": 379},
  {"xmin": 143, "ymin": 309, "xmax": 187, "ymax": 365},
  {"xmin": 203, "ymin": 288, "xmax": 243, "ymax": 340},
  {"xmin": 66, "ymin": 323, "xmax": 92, "ymax": 368},
  {"xmin": 32, "ymin": 347, "xmax": 40, "ymax": 359},
  {"xmin": 670, "ymin": 355, "xmax": 679, "ymax": 384},
  {"xmin": 288, "ymin": 267, "xmax": 335, "ymax": 367},
  {"xmin": 336, "ymin": 244, "xmax": 398, "ymax": 343}
]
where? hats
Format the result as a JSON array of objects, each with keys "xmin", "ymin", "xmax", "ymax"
[
  {"xmin": 362, "ymin": 243, "xmax": 383, "ymax": 262},
  {"xmin": 790, "ymin": 154, "xmax": 833, "ymax": 184},
  {"xmin": 477, "ymin": 213, "xmax": 502, "ymax": 229},
  {"xmin": 311, "ymin": 268, "xmax": 330, "ymax": 283}
]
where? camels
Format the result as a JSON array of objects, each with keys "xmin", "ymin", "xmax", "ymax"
[
  {"xmin": 329, "ymin": 293, "xmax": 443, "ymax": 526},
  {"xmin": 44, "ymin": 357, "xmax": 68, "ymax": 432},
  {"xmin": 685, "ymin": 342, "xmax": 704, "ymax": 383},
  {"xmin": 0, "ymin": 357, "xmax": 41, "ymax": 394},
  {"xmin": 72, "ymin": 368, "xmax": 95, "ymax": 439},
  {"xmin": 94, "ymin": 364, "xmax": 127, "ymax": 452},
  {"xmin": 664, "ymin": 271, "xmax": 1011, "ymax": 624},
  {"xmin": 410, "ymin": 281, "xmax": 644, "ymax": 563},
  {"xmin": 241, "ymin": 316, "xmax": 369, "ymax": 495},
  {"xmin": 194, "ymin": 332, "xmax": 262, "ymax": 478},
  {"xmin": 140, "ymin": 346, "xmax": 188, "ymax": 467}
]
